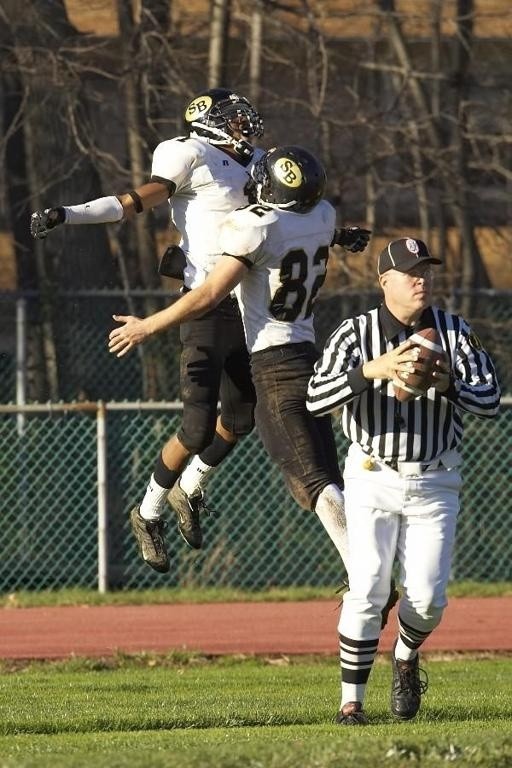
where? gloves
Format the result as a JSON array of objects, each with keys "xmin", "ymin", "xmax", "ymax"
[
  {"xmin": 30, "ymin": 206, "xmax": 66, "ymax": 239},
  {"xmin": 330, "ymin": 226, "xmax": 372, "ymax": 253}
]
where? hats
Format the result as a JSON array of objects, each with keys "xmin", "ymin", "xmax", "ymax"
[{"xmin": 377, "ymin": 237, "xmax": 442, "ymax": 276}]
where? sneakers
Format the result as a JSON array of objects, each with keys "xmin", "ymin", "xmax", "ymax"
[
  {"xmin": 391, "ymin": 637, "xmax": 428, "ymax": 718},
  {"xmin": 166, "ymin": 475, "xmax": 216, "ymax": 549},
  {"xmin": 336, "ymin": 702, "xmax": 368, "ymax": 726},
  {"xmin": 129, "ymin": 500, "xmax": 169, "ymax": 573},
  {"xmin": 332, "ymin": 575, "xmax": 399, "ymax": 631}
]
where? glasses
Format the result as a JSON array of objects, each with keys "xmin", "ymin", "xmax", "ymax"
[{"xmin": 406, "ymin": 268, "xmax": 434, "ymax": 282}]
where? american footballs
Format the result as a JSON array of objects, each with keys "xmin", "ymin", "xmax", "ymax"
[{"xmin": 392, "ymin": 328, "xmax": 445, "ymax": 403}]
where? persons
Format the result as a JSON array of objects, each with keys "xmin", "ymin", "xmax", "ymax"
[
  {"xmin": 27, "ymin": 86, "xmax": 373, "ymax": 576},
  {"xmin": 106, "ymin": 143, "xmax": 402, "ymax": 632},
  {"xmin": 302, "ymin": 236, "xmax": 503, "ymax": 724}
]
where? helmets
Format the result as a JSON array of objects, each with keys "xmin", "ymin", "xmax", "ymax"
[
  {"xmin": 182, "ymin": 88, "xmax": 264, "ymax": 162},
  {"xmin": 245, "ymin": 144, "xmax": 327, "ymax": 216}
]
